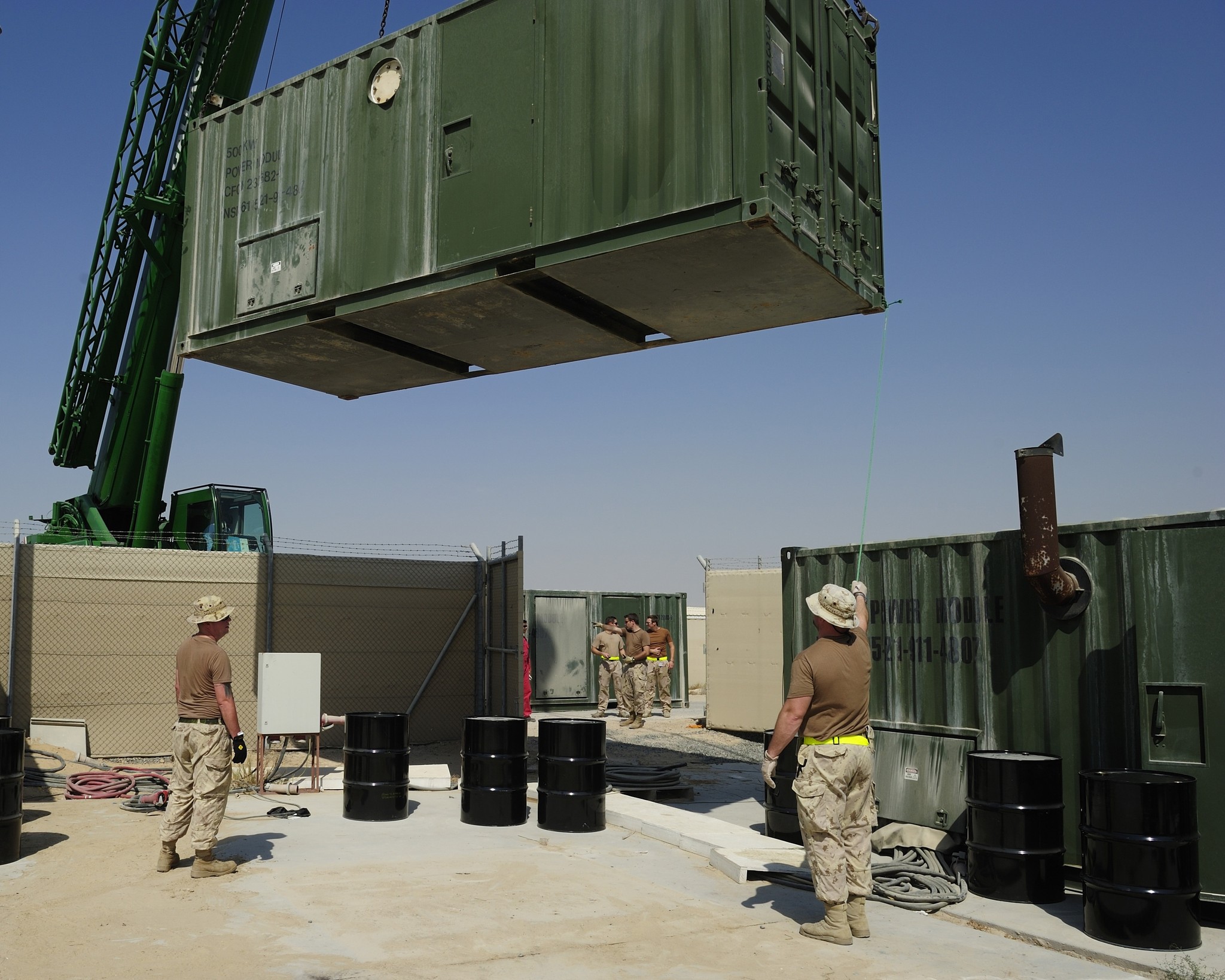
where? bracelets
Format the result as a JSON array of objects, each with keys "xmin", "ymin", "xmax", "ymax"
[{"xmin": 670, "ymin": 660, "xmax": 675, "ymax": 663}]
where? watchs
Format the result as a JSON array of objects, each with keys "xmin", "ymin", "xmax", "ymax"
[
  {"xmin": 854, "ymin": 593, "xmax": 867, "ymax": 605},
  {"xmin": 632, "ymin": 657, "xmax": 635, "ymax": 664}
]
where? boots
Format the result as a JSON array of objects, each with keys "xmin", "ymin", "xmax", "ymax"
[
  {"xmin": 628, "ymin": 712, "xmax": 643, "ymax": 729},
  {"xmin": 799, "ymin": 899, "xmax": 853, "ymax": 945},
  {"xmin": 157, "ymin": 840, "xmax": 181, "ymax": 872},
  {"xmin": 190, "ymin": 850, "xmax": 237, "ymax": 879},
  {"xmin": 846, "ymin": 895, "xmax": 870, "ymax": 939},
  {"xmin": 620, "ymin": 711, "xmax": 636, "ymax": 726}
]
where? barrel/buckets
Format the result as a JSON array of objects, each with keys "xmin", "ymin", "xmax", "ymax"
[
  {"xmin": 763, "ymin": 729, "xmax": 804, "ymax": 846},
  {"xmin": 964, "ymin": 750, "xmax": 1067, "ymax": 904},
  {"xmin": 1078, "ymin": 769, "xmax": 1203, "ymax": 952},
  {"xmin": 344, "ymin": 713, "xmax": 411, "ymax": 823},
  {"xmin": 536, "ymin": 718, "xmax": 608, "ymax": 833},
  {"xmin": 0, "ymin": 715, "xmax": 25, "ymax": 866},
  {"xmin": 459, "ymin": 716, "xmax": 529, "ymax": 827}
]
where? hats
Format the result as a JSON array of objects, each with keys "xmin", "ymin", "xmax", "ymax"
[
  {"xmin": 187, "ymin": 595, "xmax": 235, "ymax": 624},
  {"xmin": 806, "ymin": 584, "xmax": 860, "ymax": 628}
]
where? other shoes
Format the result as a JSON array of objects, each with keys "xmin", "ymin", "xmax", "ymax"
[
  {"xmin": 525, "ymin": 716, "xmax": 536, "ymax": 722},
  {"xmin": 618, "ymin": 710, "xmax": 630, "ymax": 717},
  {"xmin": 642, "ymin": 713, "xmax": 652, "ymax": 717},
  {"xmin": 663, "ymin": 711, "xmax": 670, "ymax": 717},
  {"xmin": 592, "ymin": 711, "xmax": 605, "ymax": 718}
]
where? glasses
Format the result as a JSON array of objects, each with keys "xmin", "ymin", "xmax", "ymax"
[
  {"xmin": 612, "ymin": 622, "xmax": 617, "ymax": 625},
  {"xmin": 523, "ymin": 625, "xmax": 527, "ymax": 628},
  {"xmin": 625, "ymin": 620, "xmax": 631, "ymax": 623}
]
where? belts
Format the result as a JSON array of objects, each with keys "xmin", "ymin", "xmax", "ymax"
[
  {"xmin": 646, "ymin": 656, "xmax": 667, "ymax": 661},
  {"xmin": 601, "ymin": 655, "xmax": 619, "ymax": 660},
  {"xmin": 803, "ymin": 734, "xmax": 870, "ymax": 746},
  {"xmin": 178, "ymin": 717, "xmax": 225, "ymax": 725}
]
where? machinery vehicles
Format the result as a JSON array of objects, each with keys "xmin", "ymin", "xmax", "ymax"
[{"xmin": 24, "ymin": 0, "xmax": 285, "ymax": 546}]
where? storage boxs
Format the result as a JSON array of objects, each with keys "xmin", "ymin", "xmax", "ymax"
[
  {"xmin": 781, "ymin": 509, "xmax": 1225, "ymax": 906},
  {"xmin": 523, "ymin": 589, "xmax": 690, "ymax": 713},
  {"xmin": 186, "ymin": 0, "xmax": 886, "ymax": 402}
]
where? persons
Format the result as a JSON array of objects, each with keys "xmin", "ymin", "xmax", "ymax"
[
  {"xmin": 591, "ymin": 613, "xmax": 650, "ymax": 729},
  {"xmin": 642, "ymin": 614, "xmax": 675, "ymax": 718},
  {"xmin": 761, "ymin": 580, "xmax": 878, "ymax": 946},
  {"xmin": 523, "ymin": 620, "xmax": 536, "ymax": 722},
  {"xmin": 154, "ymin": 594, "xmax": 248, "ymax": 879}
]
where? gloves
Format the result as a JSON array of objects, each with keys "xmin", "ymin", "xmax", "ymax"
[
  {"xmin": 761, "ymin": 750, "xmax": 779, "ymax": 789},
  {"xmin": 591, "ymin": 622, "xmax": 603, "ymax": 628},
  {"xmin": 622, "ymin": 656, "xmax": 633, "ymax": 664},
  {"xmin": 851, "ymin": 580, "xmax": 868, "ymax": 598},
  {"xmin": 231, "ymin": 734, "xmax": 247, "ymax": 764}
]
